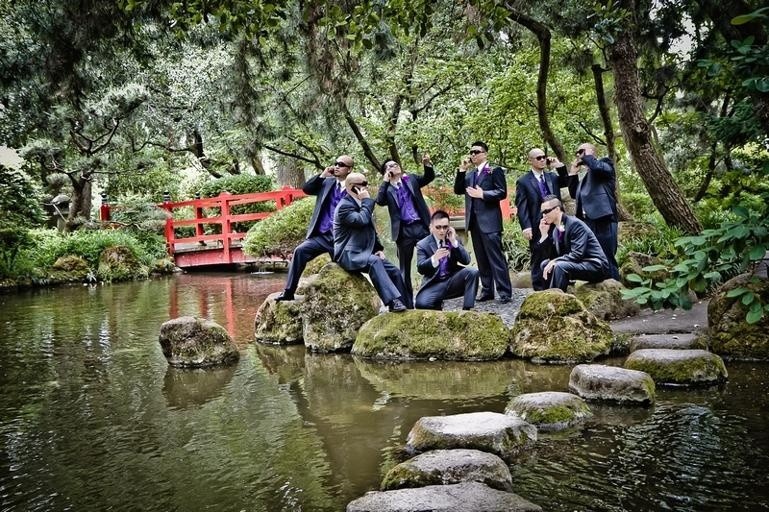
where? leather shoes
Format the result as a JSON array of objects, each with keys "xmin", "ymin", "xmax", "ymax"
[
  {"xmin": 390, "ymin": 300, "xmax": 407, "ymax": 312},
  {"xmin": 275, "ymin": 294, "xmax": 295, "ymax": 300},
  {"xmin": 475, "ymin": 294, "xmax": 510, "ymax": 303}
]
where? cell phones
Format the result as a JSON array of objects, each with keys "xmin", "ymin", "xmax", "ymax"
[
  {"xmin": 331, "ymin": 169, "xmax": 334, "ymax": 174},
  {"xmin": 388, "ymin": 172, "xmax": 394, "ymax": 177},
  {"xmin": 546, "ymin": 159, "xmax": 550, "ymax": 165},
  {"xmin": 446, "ymin": 231, "xmax": 449, "ymax": 238},
  {"xmin": 464, "ymin": 158, "xmax": 472, "ymax": 166},
  {"xmin": 351, "ymin": 186, "xmax": 360, "ymax": 194}
]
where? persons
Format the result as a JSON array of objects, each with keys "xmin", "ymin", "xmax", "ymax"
[
  {"xmin": 567, "ymin": 143, "xmax": 618, "ymax": 280},
  {"xmin": 374, "ymin": 149, "xmax": 436, "ymax": 310},
  {"xmin": 514, "ymin": 148, "xmax": 569, "ymax": 292},
  {"xmin": 534, "ymin": 194, "xmax": 610, "ymax": 291},
  {"xmin": 333, "ymin": 171, "xmax": 414, "ymax": 312},
  {"xmin": 453, "ymin": 141, "xmax": 512, "ymax": 305},
  {"xmin": 273, "ymin": 154, "xmax": 355, "ymax": 302},
  {"xmin": 415, "ymin": 210, "xmax": 479, "ymax": 309}
]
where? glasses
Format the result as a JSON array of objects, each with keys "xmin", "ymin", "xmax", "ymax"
[
  {"xmin": 355, "ymin": 181, "xmax": 367, "ymax": 186},
  {"xmin": 470, "ymin": 150, "xmax": 484, "ymax": 155},
  {"xmin": 543, "ymin": 206, "xmax": 556, "ymax": 214},
  {"xmin": 536, "ymin": 156, "xmax": 546, "ymax": 160},
  {"xmin": 576, "ymin": 149, "xmax": 584, "ymax": 154},
  {"xmin": 436, "ymin": 225, "xmax": 448, "ymax": 229},
  {"xmin": 335, "ymin": 162, "xmax": 351, "ymax": 168}
]
